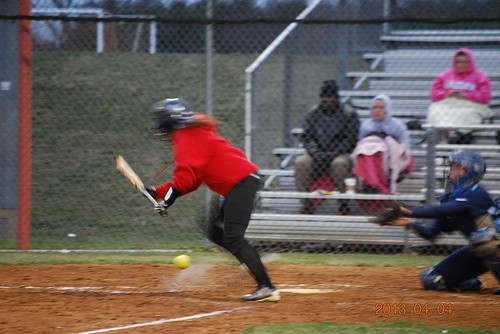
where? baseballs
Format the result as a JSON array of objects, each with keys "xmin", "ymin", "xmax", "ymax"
[{"xmin": 174, "ymin": 255, "xmax": 192, "ymax": 269}]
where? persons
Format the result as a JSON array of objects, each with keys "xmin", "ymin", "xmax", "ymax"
[
  {"xmin": 350, "ymin": 94, "xmax": 415, "ymax": 213},
  {"xmin": 424, "ymin": 47, "xmax": 493, "ymax": 144},
  {"xmin": 368, "ymin": 148, "xmax": 500, "ymax": 295},
  {"xmin": 294, "ymin": 80, "xmax": 360, "ymax": 214},
  {"xmin": 130, "ymin": 97, "xmax": 281, "ymax": 302}
]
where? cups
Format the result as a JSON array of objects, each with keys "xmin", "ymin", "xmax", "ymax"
[{"xmin": 344, "ymin": 178, "xmax": 356, "ymax": 194}]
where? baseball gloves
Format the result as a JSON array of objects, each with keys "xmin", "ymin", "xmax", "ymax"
[{"xmin": 370, "ymin": 202, "xmax": 414, "ymax": 227}]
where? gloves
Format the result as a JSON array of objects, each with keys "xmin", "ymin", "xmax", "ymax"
[
  {"xmin": 153, "ymin": 202, "xmax": 169, "ymax": 217},
  {"xmin": 142, "ymin": 185, "xmax": 157, "ymax": 199}
]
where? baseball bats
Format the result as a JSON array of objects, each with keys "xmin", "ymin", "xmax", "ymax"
[{"xmin": 116, "ymin": 154, "xmax": 166, "ymax": 211}]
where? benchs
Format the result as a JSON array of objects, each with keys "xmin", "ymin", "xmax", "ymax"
[{"xmin": 245, "ymin": 30, "xmax": 500, "ymax": 244}]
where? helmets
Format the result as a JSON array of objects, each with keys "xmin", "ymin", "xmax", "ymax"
[
  {"xmin": 149, "ymin": 97, "xmax": 191, "ymax": 118},
  {"xmin": 447, "ymin": 149, "xmax": 487, "ymax": 180}
]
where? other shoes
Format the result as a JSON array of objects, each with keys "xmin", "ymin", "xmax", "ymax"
[
  {"xmin": 337, "ymin": 202, "xmax": 356, "ymax": 215},
  {"xmin": 239, "ymin": 286, "xmax": 281, "ymax": 302},
  {"xmin": 299, "ymin": 203, "xmax": 315, "ymax": 214}
]
